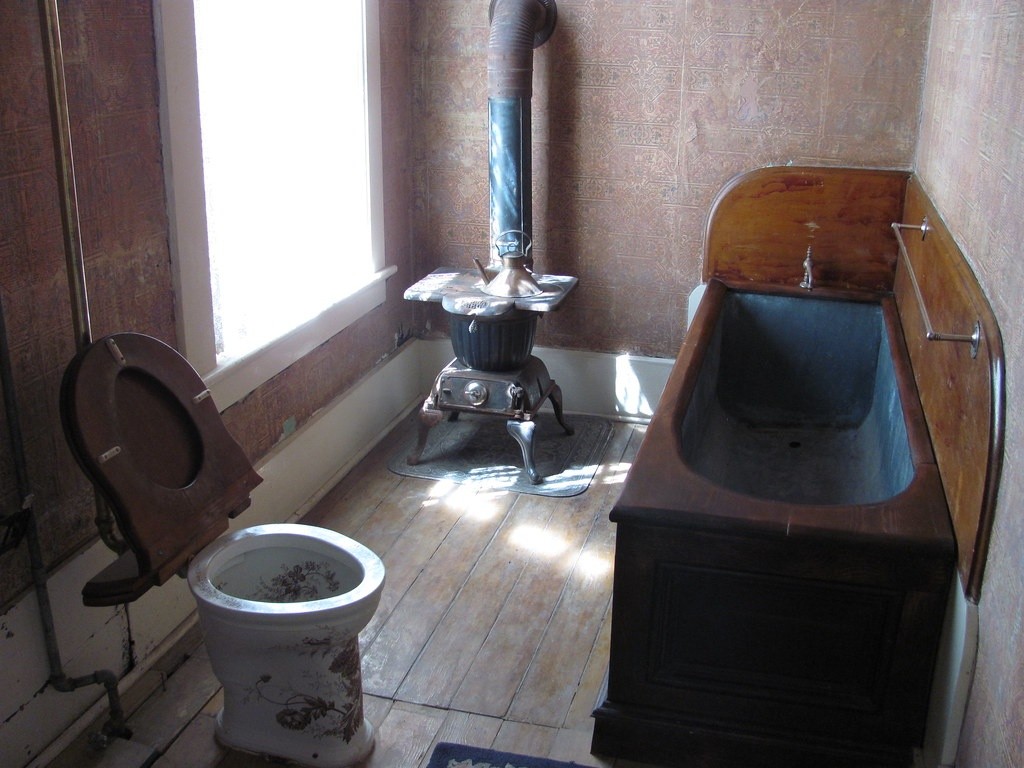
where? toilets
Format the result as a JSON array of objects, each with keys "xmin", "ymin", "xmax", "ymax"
[{"xmin": 58, "ymin": 330, "xmax": 389, "ymax": 768}]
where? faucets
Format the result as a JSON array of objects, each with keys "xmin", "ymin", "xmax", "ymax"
[{"xmin": 799, "ymin": 243, "xmax": 818, "ymax": 291}]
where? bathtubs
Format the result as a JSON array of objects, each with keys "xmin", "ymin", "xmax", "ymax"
[{"xmin": 589, "ymin": 274, "xmax": 957, "ymax": 752}]
